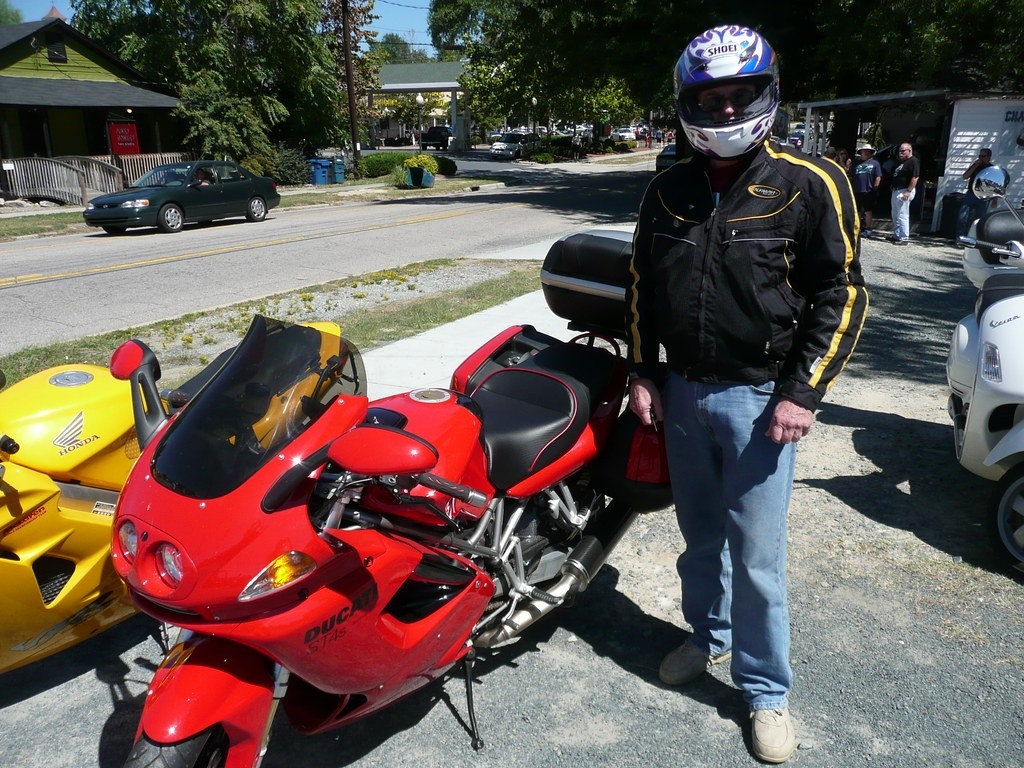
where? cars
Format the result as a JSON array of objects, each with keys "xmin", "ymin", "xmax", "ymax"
[
  {"xmin": 489, "ymin": 132, "xmax": 542, "ymax": 163},
  {"xmin": 472, "ymin": 122, "xmax": 655, "ymax": 142},
  {"xmin": 83, "ymin": 160, "xmax": 281, "ymax": 236},
  {"xmin": 771, "ymin": 123, "xmax": 815, "ymax": 152},
  {"xmin": 656, "ymin": 144, "xmax": 676, "ymax": 173}
]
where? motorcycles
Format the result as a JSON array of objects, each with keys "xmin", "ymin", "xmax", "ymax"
[
  {"xmin": 942, "ymin": 164, "xmax": 1023, "ymax": 577},
  {"xmin": 1, "ymin": 321, "xmax": 352, "ymax": 676},
  {"xmin": 110, "ymin": 228, "xmax": 678, "ymax": 768}
]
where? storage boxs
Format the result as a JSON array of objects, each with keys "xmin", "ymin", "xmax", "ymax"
[
  {"xmin": 594, "ymin": 362, "xmax": 674, "ymax": 515},
  {"xmin": 540, "ymin": 230, "xmax": 634, "ymax": 332},
  {"xmin": 962, "ymin": 208, "xmax": 1024, "ymax": 288},
  {"xmin": 451, "ymin": 324, "xmax": 565, "ymax": 397}
]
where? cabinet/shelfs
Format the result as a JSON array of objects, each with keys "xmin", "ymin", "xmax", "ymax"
[{"xmin": 920, "ymin": 182, "xmax": 937, "ymax": 221}]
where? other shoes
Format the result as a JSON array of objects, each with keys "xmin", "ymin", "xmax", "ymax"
[
  {"xmin": 865, "ymin": 230, "xmax": 872, "ymax": 238},
  {"xmin": 886, "ymin": 235, "xmax": 897, "ymax": 241},
  {"xmin": 894, "ymin": 239, "xmax": 908, "ymax": 246}
]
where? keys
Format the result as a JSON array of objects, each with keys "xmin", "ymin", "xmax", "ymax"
[{"xmin": 651, "ymin": 411, "xmax": 660, "ymax": 432}]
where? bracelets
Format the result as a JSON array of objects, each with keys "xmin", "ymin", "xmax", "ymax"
[{"xmin": 907, "ymin": 189, "xmax": 911, "ymax": 192}]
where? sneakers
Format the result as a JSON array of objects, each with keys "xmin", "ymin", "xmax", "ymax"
[
  {"xmin": 749, "ymin": 700, "xmax": 792, "ymax": 764},
  {"xmin": 659, "ymin": 632, "xmax": 734, "ymax": 686}
]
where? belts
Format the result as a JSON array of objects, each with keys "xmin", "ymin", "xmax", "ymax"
[{"xmin": 894, "ymin": 188, "xmax": 905, "ymax": 190}]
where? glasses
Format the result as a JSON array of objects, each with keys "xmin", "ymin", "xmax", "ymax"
[
  {"xmin": 701, "ymin": 87, "xmax": 755, "ymax": 114},
  {"xmin": 899, "ymin": 148, "xmax": 909, "ymax": 153},
  {"xmin": 978, "ymin": 154, "xmax": 989, "ymax": 158}
]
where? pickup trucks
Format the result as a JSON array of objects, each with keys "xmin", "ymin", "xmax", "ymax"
[{"xmin": 419, "ymin": 126, "xmax": 453, "ymax": 151}]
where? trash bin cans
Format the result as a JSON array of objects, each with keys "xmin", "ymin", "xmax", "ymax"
[
  {"xmin": 941, "ymin": 192, "xmax": 987, "ymax": 240},
  {"xmin": 330, "ymin": 156, "xmax": 344, "ymax": 184},
  {"xmin": 406, "ymin": 164, "xmax": 435, "ymax": 188},
  {"xmin": 307, "ymin": 159, "xmax": 330, "ymax": 185}
]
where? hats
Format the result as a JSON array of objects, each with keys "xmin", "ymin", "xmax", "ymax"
[{"xmin": 856, "ymin": 144, "xmax": 875, "ymax": 156}]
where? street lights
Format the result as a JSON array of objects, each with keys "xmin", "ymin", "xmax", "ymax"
[
  {"xmin": 384, "ymin": 106, "xmax": 390, "ymax": 138},
  {"xmin": 416, "ymin": 93, "xmax": 424, "ymax": 155}
]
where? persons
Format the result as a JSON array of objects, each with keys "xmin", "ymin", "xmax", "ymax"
[
  {"xmin": 625, "ymin": 25, "xmax": 869, "ymax": 762},
  {"xmin": 191, "ymin": 168, "xmax": 212, "ymax": 185},
  {"xmin": 157, "ymin": 171, "xmax": 177, "ymax": 185},
  {"xmin": 885, "ymin": 142, "xmax": 919, "ymax": 246},
  {"xmin": 956, "ymin": 149, "xmax": 999, "ymax": 248},
  {"xmin": 572, "ymin": 134, "xmax": 583, "ymax": 162},
  {"xmin": 636, "ymin": 127, "xmax": 674, "ymax": 148},
  {"xmin": 807, "ymin": 144, "xmax": 882, "ymax": 237}
]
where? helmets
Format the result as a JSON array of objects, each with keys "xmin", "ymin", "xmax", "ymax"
[{"xmin": 673, "ymin": 24, "xmax": 778, "ymax": 159}]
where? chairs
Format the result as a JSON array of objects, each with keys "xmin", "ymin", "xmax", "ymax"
[{"xmin": 204, "ymin": 172, "xmax": 213, "ymax": 184}]
